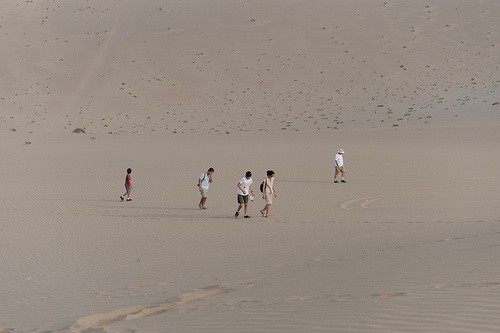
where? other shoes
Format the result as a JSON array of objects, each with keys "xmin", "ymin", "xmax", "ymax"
[
  {"xmin": 244, "ymin": 215, "xmax": 250, "ymax": 218},
  {"xmin": 341, "ymin": 180, "xmax": 346, "ymax": 183},
  {"xmin": 203, "ymin": 208, "xmax": 207, "ymax": 209},
  {"xmin": 127, "ymin": 198, "xmax": 132, "ymax": 201},
  {"xmin": 235, "ymin": 211, "xmax": 239, "ymax": 217},
  {"xmin": 260, "ymin": 210, "xmax": 265, "ymax": 216},
  {"xmin": 199, "ymin": 204, "xmax": 202, "ymax": 210},
  {"xmin": 334, "ymin": 180, "xmax": 339, "ymax": 183},
  {"xmin": 120, "ymin": 196, "xmax": 124, "ymax": 201}
]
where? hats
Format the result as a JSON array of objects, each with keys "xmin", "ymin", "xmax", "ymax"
[{"xmin": 337, "ymin": 148, "xmax": 345, "ymax": 153}]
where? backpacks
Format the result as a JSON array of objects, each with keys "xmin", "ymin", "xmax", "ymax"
[{"xmin": 259, "ymin": 178, "xmax": 268, "ymax": 193}]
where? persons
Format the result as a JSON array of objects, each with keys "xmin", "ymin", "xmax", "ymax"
[
  {"xmin": 235, "ymin": 171, "xmax": 254, "ymax": 218},
  {"xmin": 334, "ymin": 149, "xmax": 346, "ymax": 183},
  {"xmin": 120, "ymin": 168, "xmax": 132, "ymax": 201},
  {"xmin": 197, "ymin": 167, "xmax": 214, "ymax": 210},
  {"xmin": 260, "ymin": 170, "xmax": 277, "ymax": 217}
]
